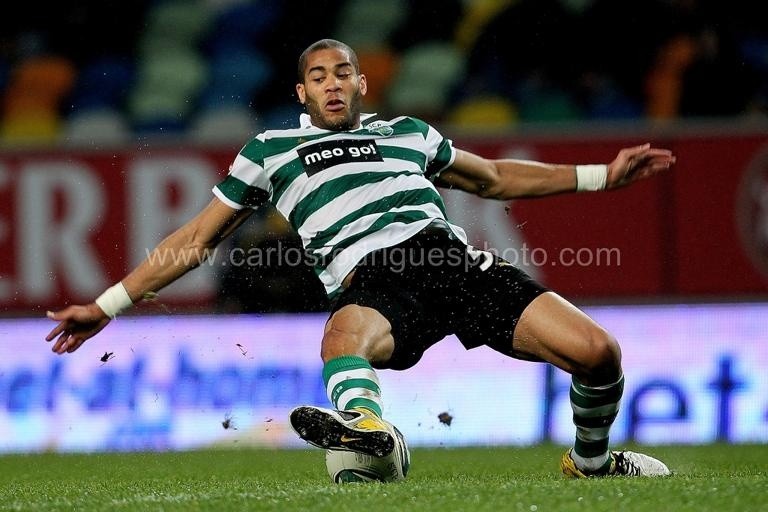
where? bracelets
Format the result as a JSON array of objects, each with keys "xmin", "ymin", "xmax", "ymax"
[
  {"xmin": 96, "ymin": 282, "xmax": 136, "ymax": 319},
  {"xmin": 575, "ymin": 164, "xmax": 608, "ymax": 192}
]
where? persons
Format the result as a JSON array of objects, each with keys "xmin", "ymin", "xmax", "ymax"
[{"xmin": 45, "ymin": 38, "xmax": 676, "ymax": 477}]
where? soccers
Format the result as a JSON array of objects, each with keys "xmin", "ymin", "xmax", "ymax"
[{"xmin": 325, "ymin": 420, "xmax": 410, "ymax": 481}]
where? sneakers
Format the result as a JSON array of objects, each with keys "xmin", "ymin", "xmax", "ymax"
[
  {"xmin": 290, "ymin": 404, "xmax": 395, "ymax": 458},
  {"xmin": 560, "ymin": 447, "xmax": 670, "ymax": 477}
]
